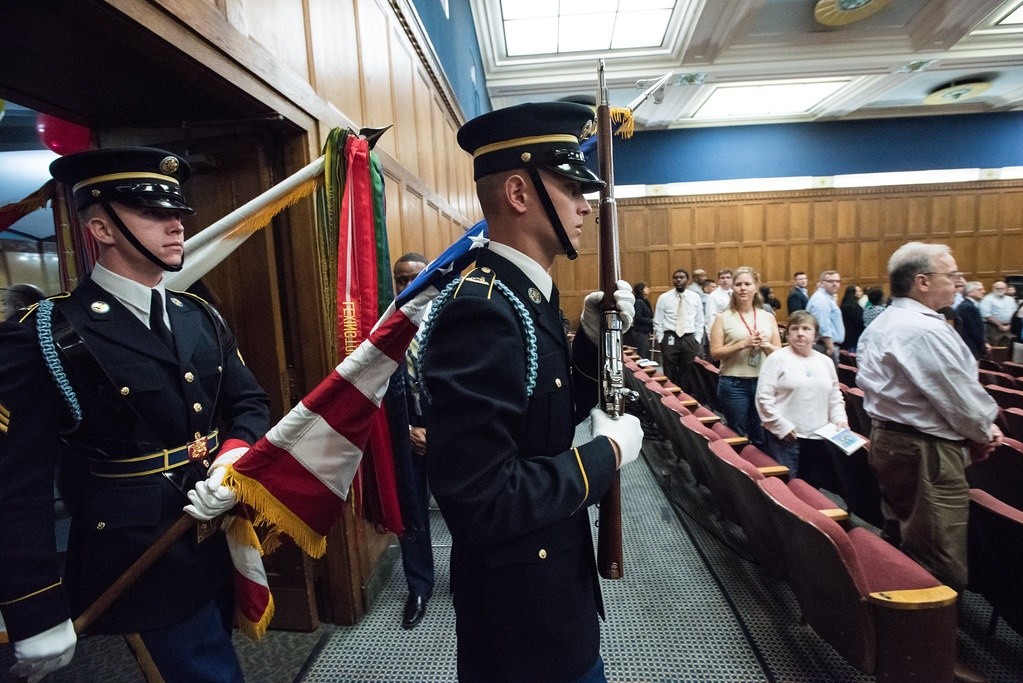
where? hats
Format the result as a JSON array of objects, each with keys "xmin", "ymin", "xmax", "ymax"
[
  {"xmin": 457, "ymin": 101, "xmax": 606, "ymax": 193},
  {"xmin": 49, "ymin": 145, "xmax": 196, "ymax": 216}
]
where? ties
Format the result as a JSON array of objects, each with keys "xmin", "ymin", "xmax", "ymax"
[
  {"xmin": 675, "ymin": 293, "xmax": 685, "ymax": 338},
  {"xmin": 149, "ymin": 286, "xmax": 177, "ymax": 358},
  {"xmin": 551, "ymin": 278, "xmax": 561, "ymax": 318}
]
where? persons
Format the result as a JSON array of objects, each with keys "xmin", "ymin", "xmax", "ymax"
[
  {"xmin": 417, "ymin": 101, "xmax": 643, "ymax": 683},
  {"xmin": 382, "ymin": 252, "xmax": 452, "ymax": 628},
  {"xmin": 1, "ymin": 284, "xmax": 45, "ymax": 320},
  {"xmin": 755, "ymin": 309, "xmax": 850, "ymax": 490},
  {"xmin": 623, "ymin": 268, "xmax": 1023, "ymax": 392},
  {"xmin": 0, "ymin": 145, "xmax": 271, "ymax": 683},
  {"xmin": 853, "ymin": 241, "xmax": 1002, "ymax": 683},
  {"xmin": 710, "ymin": 265, "xmax": 782, "ymax": 450}
]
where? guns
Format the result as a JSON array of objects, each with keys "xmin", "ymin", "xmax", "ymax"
[{"xmin": 594, "ymin": 53, "xmax": 641, "ymax": 582}]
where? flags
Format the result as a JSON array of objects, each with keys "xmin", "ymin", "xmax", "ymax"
[{"xmin": 216, "ymin": 105, "xmax": 633, "ymax": 643}]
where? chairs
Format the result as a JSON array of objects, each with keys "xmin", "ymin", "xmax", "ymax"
[{"xmin": 620, "ymin": 345, "xmax": 1023, "ymax": 683}]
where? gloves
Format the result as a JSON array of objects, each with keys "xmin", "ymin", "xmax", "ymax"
[
  {"xmin": 589, "ymin": 408, "xmax": 645, "ymax": 468},
  {"xmin": 183, "ymin": 465, "xmax": 239, "ymax": 522},
  {"xmin": 580, "ymin": 281, "xmax": 636, "ymax": 345},
  {"xmin": 9, "ymin": 618, "xmax": 77, "ymax": 683}
]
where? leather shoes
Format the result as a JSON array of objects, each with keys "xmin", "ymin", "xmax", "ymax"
[{"xmin": 402, "ymin": 593, "xmax": 429, "ymax": 627}]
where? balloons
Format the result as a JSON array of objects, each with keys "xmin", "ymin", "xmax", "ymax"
[{"xmin": 36, "ymin": 112, "xmax": 92, "ymax": 154}]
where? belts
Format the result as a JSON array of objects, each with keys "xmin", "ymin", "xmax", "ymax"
[{"xmin": 870, "ymin": 418, "xmax": 920, "ymax": 433}]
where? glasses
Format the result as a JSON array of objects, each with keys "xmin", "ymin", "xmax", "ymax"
[
  {"xmin": 823, "ymin": 280, "xmax": 841, "ymax": 284},
  {"xmin": 922, "ymin": 272, "xmax": 961, "ymax": 282}
]
